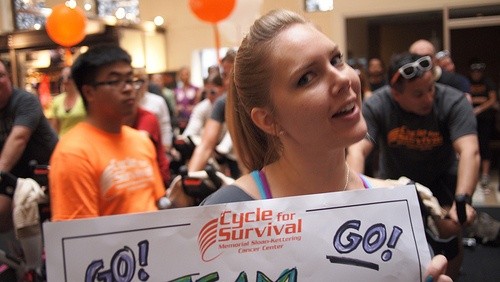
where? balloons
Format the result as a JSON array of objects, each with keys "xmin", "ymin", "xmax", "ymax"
[
  {"xmin": 188, "ymin": 0, "xmax": 237, "ymax": 23},
  {"xmin": 46, "ymin": 4, "xmax": 88, "ymax": 46}
]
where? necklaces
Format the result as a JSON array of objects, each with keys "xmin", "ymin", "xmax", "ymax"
[{"xmin": 278, "ymin": 159, "xmax": 349, "ymax": 194}]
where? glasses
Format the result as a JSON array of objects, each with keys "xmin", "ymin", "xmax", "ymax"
[
  {"xmin": 390, "ymin": 56, "xmax": 432, "ymax": 87},
  {"xmin": 90, "ymin": 77, "xmax": 145, "ymax": 90},
  {"xmin": 204, "ymin": 89, "xmax": 220, "ymax": 95}
]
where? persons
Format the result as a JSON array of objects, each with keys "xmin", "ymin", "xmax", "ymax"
[
  {"xmin": 42, "ymin": 65, "xmax": 87, "ymax": 137},
  {"xmin": 126, "ymin": 39, "xmax": 500, "ymax": 209},
  {"xmin": 48, "ymin": 45, "xmax": 172, "ymax": 222},
  {"xmin": 200, "ymin": 8, "xmax": 453, "ymax": 282},
  {"xmin": 0, "ymin": 57, "xmax": 59, "ymax": 282},
  {"xmin": 343, "ymin": 51, "xmax": 482, "ymax": 282}
]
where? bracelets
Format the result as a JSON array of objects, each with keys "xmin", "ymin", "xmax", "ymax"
[{"xmin": 454, "ymin": 193, "xmax": 473, "ymax": 206}]
